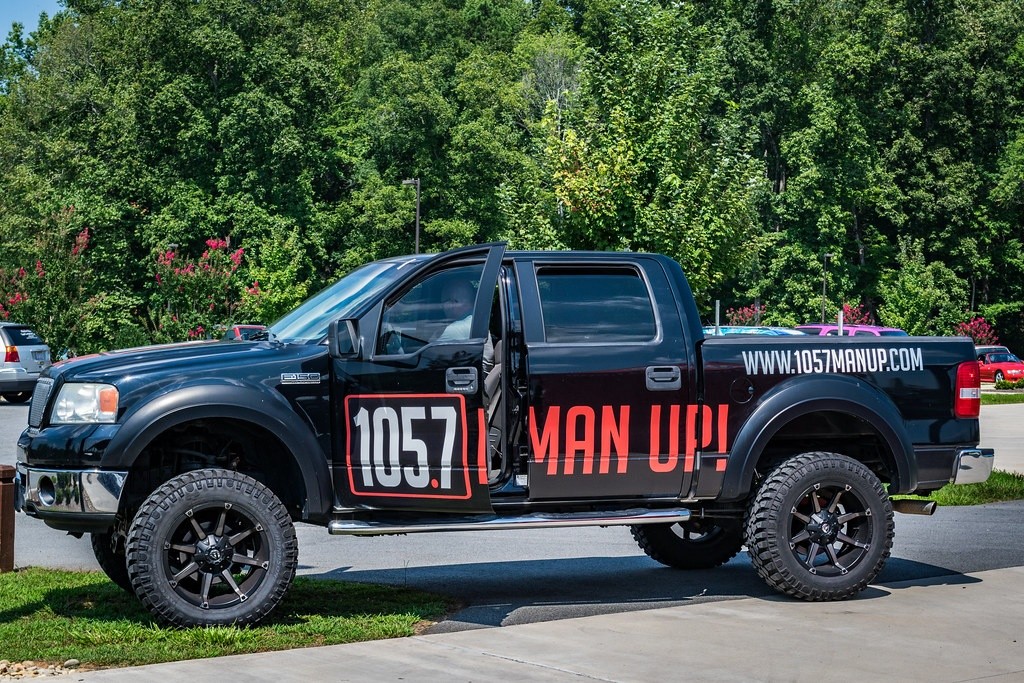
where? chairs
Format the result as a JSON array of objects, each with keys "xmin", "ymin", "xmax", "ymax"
[{"xmin": 543, "ymin": 278, "xmax": 586, "ymax": 302}]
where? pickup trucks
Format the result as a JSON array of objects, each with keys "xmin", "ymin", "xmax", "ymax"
[{"xmin": 22, "ymin": 239, "xmax": 995, "ymax": 630}]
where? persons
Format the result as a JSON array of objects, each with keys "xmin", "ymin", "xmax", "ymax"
[{"xmin": 435, "ymin": 277, "xmax": 495, "ymax": 382}]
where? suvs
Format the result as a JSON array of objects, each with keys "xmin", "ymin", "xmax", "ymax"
[{"xmin": 0, "ymin": 320, "xmax": 52, "ymax": 404}]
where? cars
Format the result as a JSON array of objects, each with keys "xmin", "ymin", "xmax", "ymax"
[
  {"xmin": 225, "ymin": 323, "xmax": 267, "ymax": 340},
  {"xmin": 701, "ymin": 321, "xmax": 910, "ymax": 337},
  {"xmin": 975, "ymin": 345, "xmax": 1024, "ymax": 386}
]
